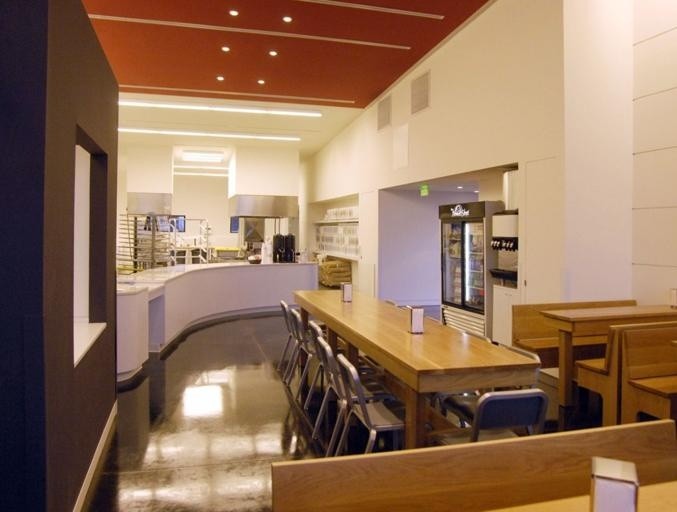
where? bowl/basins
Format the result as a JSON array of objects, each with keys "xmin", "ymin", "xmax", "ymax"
[{"xmin": 249, "ymin": 260, "xmax": 261, "ymax": 263}]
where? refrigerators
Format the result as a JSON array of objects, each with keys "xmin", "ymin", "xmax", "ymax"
[{"xmin": 439, "ymin": 201, "xmax": 505, "ymax": 343}]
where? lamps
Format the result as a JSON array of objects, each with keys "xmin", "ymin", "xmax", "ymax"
[
  {"xmin": 116, "ymin": 125, "xmax": 301, "ymax": 141},
  {"xmin": 118, "ymin": 98, "xmax": 324, "ymax": 118}
]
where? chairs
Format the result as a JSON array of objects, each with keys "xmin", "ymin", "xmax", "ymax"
[{"xmin": 277, "ymin": 299, "xmax": 550, "ymax": 458}]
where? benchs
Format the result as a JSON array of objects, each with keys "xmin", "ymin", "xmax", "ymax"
[
  {"xmin": 575, "ymin": 321, "xmax": 676, "ymax": 426},
  {"xmin": 619, "ymin": 326, "xmax": 676, "ymax": 424},
  {"xmin": 513, "ymin": 299, "xmax": 638, "ymax": 367}
]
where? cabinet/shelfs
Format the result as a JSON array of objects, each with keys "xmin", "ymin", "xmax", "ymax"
[{"xmin": 312, "ymin": 220, "xmax": 359, "ymax": 262}]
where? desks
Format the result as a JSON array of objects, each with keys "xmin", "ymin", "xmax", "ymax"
[{"xmin": 293, "ymin": 288, "xmax": 543, "ymax": 448}]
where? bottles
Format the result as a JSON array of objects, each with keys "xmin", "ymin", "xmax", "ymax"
[
  {"xmin": 144, "ymin": 216, "xmax": 151, "ymax": 231},
  {"xmin": 262, "ymin": 240, "xmax": 273, "ymax": 264}
]
